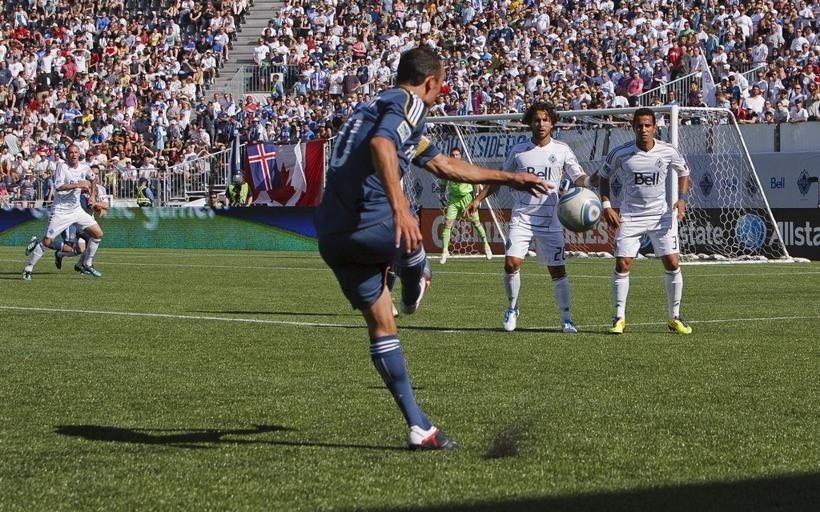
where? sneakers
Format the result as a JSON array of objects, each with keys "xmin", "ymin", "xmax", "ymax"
[
  {"xmin": 668, "ymin": 315, "xmax": 692, "ymax": 334},
  {"xmin": 485, "ymin": 248, "xmax": 492, "ymax": 259},
  {"xmin": 74, "ymin": 263, "xmax": 102, "ymax": 277},
  {"xmin": 562, "ymin": 320, "xmax": 578, "ymax": 333},
  {"xmin": 25, "ymin": 239, "xmax": 40, "ymax": 256},
  {"xmin": 440, "ymin": 253, "xmax": 450, "ymax": 264},
  {"xmin": 407, "ymin": 425, "xmax": 459, "ymax": 451},
  {"xmin": 607, "ymin": 317, "xmax": 626, "ymax": 334},
  {"xmin": 400, "ymin": 258, "xmax": 432, "ymax": 314},
  {"xmin": 55, "ymin": 250, "xmax": 63, "ymax": 269},
  {"xmin": 22, "ymin": 267, "xmax": 32, "ymax": 280},
  {"xmin": 502, "ymin": 305, "xmax": 520, "ymax": 331}
]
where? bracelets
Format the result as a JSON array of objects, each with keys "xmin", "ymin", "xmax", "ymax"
[
  {"xmin": 678, "ymin": 192, "xmax": 689, "ymax": 202},
  {"xmin": 602, "ymin": 200, "xmax": 612, "ymax": 211},
  {"xmin": 582, "ymin": 175, "xmax": 592, "ymax": 187}
]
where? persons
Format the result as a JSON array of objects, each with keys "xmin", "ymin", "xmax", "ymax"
[
  {"xmin": 596, "ymin": 109, "xmax": 694, "ymax": 335},
  {"xmin": 458, "ymin": 100, "xmax": 602, "ymax": 334},
  {"xmin": 0, "ymin": 1, "xmax": 819, "ymax": 213},
  {"xmin": 436, "ymin": 146, "xmax": 496, "ymax": 265},
  {"xmin": 22, "ymin": 145, "xmax": 103, "ymax": 279},
  {"xmin": 310, "ymin": 44, "xmax": 556, "ymax": 452},
  {"xmin": 24, "ymin": 163, "xmax": 110, "ymax": 270}
]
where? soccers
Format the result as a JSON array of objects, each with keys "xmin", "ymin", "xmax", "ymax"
[{"xmin": 557, "ymin": 186, "xmax": 602, "ymax": 232}]
